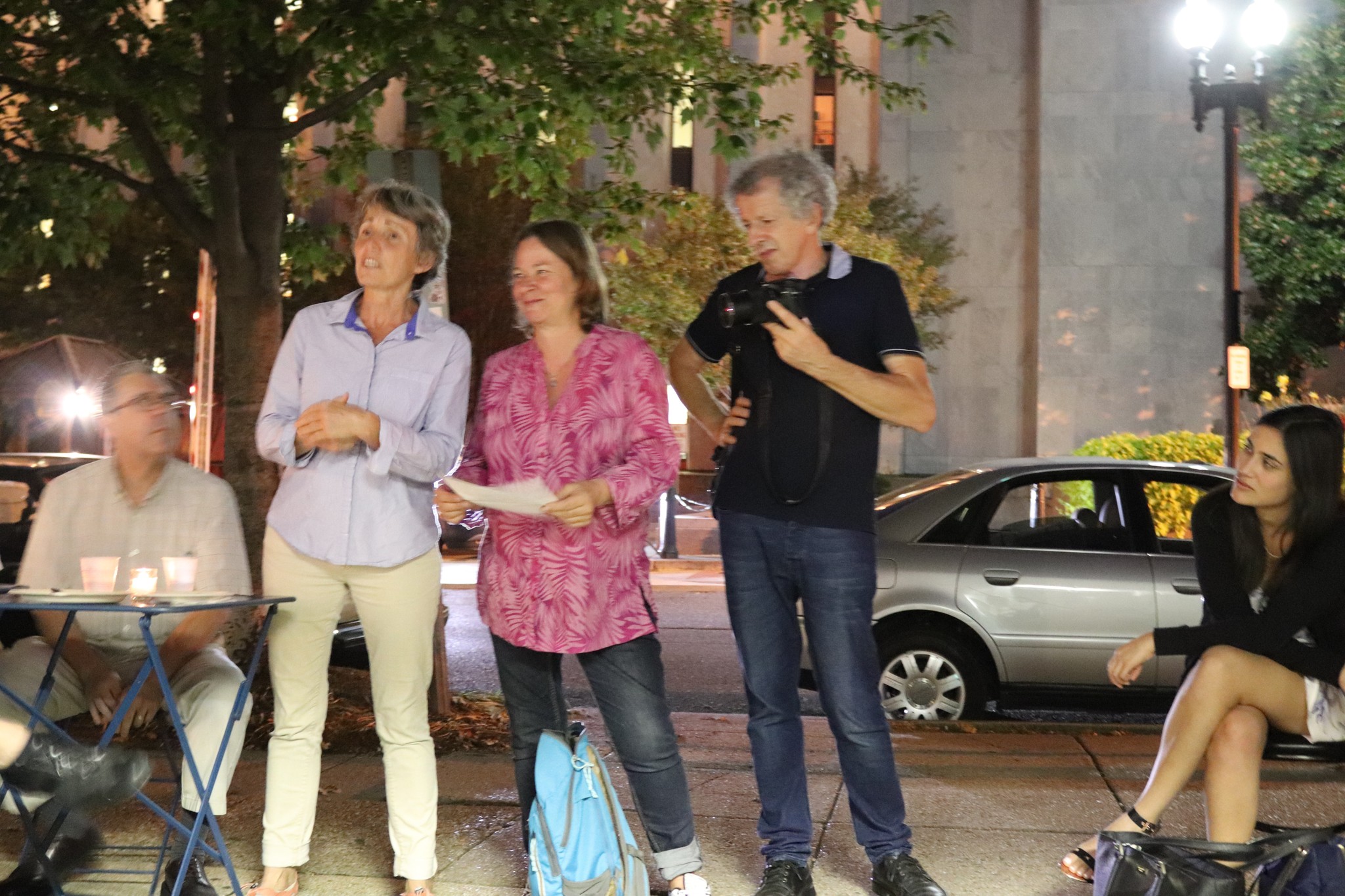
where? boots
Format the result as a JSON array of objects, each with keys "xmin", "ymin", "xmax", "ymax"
[{"xmin": 0, "ymin": 732, "xmax": 151, "ymax": 809}]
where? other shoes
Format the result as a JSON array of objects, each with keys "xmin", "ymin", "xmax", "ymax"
[
  {"xmin": 240, "ymin": 870, "xmax": 299, "ymax": 896},
  {"xmin": 400, "ymin": 886, "xmax": 433, "ymax": 896}
]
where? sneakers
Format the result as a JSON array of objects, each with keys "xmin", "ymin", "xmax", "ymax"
[
  {"xmin": 755, "ymin": 857, "xmax": 816, "ymax": 896},
  {"xmin": 668, "ymin": 872, "xmax": 712, "ymax": 896},
  {"xmin": 871, "ymin": 850, "xmax": 947, "ymax": 896}
]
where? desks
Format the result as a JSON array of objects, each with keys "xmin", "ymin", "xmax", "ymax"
[{"xmin": 0, "ymin": 592, "xmax": 297, "ymax": 896}]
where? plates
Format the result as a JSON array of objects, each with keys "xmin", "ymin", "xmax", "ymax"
[
  {"xmin": 9, "ymin": 589, "xmax": 128, "ymax": 603},
  {"xmin": 135, "ymin": 594, "xmax": 229, "ymax": 604}
]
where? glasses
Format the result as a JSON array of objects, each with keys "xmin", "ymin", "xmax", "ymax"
[{"xmin": 110, "ymin": 392, "xmax": 180, "ymax": 413}]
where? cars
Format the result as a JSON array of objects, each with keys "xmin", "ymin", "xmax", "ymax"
[
  {"xmin": 0, "ymin": 451, "xmax": 112, "ymax": 640},
  {"xmin": 798, "ymin": 459, "xmax": 1309, "ymax": 721}
]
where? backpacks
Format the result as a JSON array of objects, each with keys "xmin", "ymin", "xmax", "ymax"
[{"xmin": 527, "ymin": 720, "xmax": 651, "ymax": 895}]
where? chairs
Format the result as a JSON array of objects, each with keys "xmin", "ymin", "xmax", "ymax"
[
  {"xmin": 1070, "ymin": 496, "xmax": 1121, "ymax": 530},
  {"xmin": 1171, "ymin": 577, "xmax": 1345, "ymax": 834}
]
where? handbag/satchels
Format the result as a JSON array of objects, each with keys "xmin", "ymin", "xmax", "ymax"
[
  {"xmin": 1244, "ymin": 832, "xmax": 1345, "ymax": 896},
  {"xmin": 1093, "ymin": 828, "xmax": 1335, "ymax": 896}
]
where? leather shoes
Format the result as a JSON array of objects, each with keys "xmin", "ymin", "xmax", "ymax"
[
  {"xmin": 7, "ymin": 825, "xmax": 101, "ymax": 896},
  {"xmin": 161, "ymin": 855, "xmax": 217, "ymax": 896}
]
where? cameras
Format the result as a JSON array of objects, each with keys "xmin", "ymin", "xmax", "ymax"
[{"xmin": 716, "ymin": 280, "xmax": 802, "ymax": 332}]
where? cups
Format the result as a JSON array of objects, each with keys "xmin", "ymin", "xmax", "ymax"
[
  {"xmin": 81, "ymin": 556, "xmax": 120, "ymax": 594},
  {"xmin": 161, "ymin": 557, "xmax": 198, "ymax": 594},
  {"xmin": 130, "ymin": 568, "xmax": 158, "ymax": 608}
]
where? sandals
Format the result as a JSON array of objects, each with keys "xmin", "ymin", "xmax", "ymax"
[{"xmin": 1062, "ymin": 806, "xmax": 1162, "ymax": 885}]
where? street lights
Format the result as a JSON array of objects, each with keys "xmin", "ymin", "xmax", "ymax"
[{"xmin": 1170, "ymin": 1, "xmax": 1288, "ymax": 469}]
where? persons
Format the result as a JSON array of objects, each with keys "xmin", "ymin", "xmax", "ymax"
[
  {"xmin": 0, "ymin": 359, "xmax": 253, "ymax": 896},
  {"xmin": 666, "ymin": 149, "xmax": 947, "ymax": 896},
  {"xmin": 1060, "ymin": 404, "xmax": 1344, "ymax": 884},
  {"xmin": 432, "ymin": 222, "xmax": 709, "ymax": 895},
  {"xmin": 240, "ymin": 186, "xmax": 472, "ymax": 896}
]
